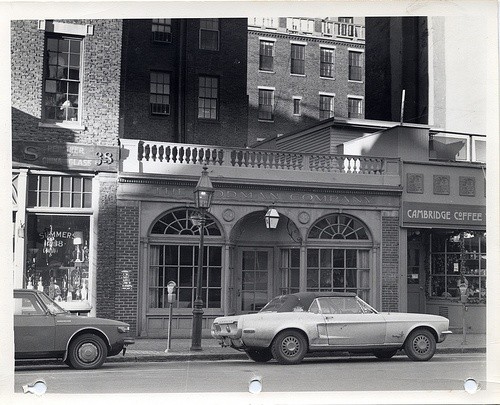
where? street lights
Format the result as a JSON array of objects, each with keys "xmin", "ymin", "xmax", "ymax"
[{"xmin": 189, "ymin": 163, "xmax": 215, "ymax": 351}]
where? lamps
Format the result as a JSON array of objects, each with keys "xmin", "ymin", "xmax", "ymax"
[
  {"xmin": 29, "ymin": 248, "xmax": 40, "ymax": 285},
  {"xmin": 72, "ymin": 230, "xmax": 83, "ymax": 263},
  {"xmin": 264, "ymin": 201, "xmax": 281, "ymax": 230}
]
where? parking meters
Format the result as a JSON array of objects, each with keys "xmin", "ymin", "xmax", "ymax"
[
  {"xmin": 163, "ymin": 281, "xmax": 177, "ymax": 354},
  {"xmin": 459, "ymin": 286, "xmax": 470, "ymax": 345}
]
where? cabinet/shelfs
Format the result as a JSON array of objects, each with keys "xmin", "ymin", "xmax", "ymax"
[{"xmin": 427, "ymin": 231, "xmax": 486, "ymax": 301}]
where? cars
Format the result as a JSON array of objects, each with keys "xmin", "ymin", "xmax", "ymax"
[
  {"xmin": 210, "ymin": 291, "xmax": 454, "ymax": 365},
  {"xmin": 13, "ymin": 288, "xmax": 135, "ymax": 370}
]
